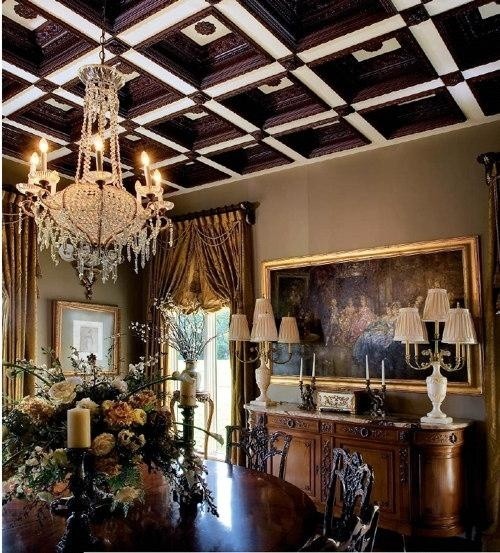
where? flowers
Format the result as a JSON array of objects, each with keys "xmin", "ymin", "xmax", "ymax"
[
  {"xmin": 128, "ymin": 295, "xmax": 232, "ymax": 360},
  {"xmin": 4, "ymin": 344, "xmax": 217, "ymax": 515}
]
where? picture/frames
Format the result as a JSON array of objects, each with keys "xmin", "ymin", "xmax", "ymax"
[
  {"xmin": 51, "ymin": 299, "xmax": 121, "ymax": 377},
  {"xmin": 256, "ymin": 233, "xmax": 487, "ymax": 396}
]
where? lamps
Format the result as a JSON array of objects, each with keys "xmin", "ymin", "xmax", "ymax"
[
  {"xmin": 392, "ymin": 288, "xmax": 478, "ymax": 424},
  {"xmin": 228, "ymin": 298, "xmax": 301, "ymax": 406},
  {"xmin": 13, "ymin": 0, "xmax": 176, "ymax": 302}
]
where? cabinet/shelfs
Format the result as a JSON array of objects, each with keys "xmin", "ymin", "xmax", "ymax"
[{"xmin": 263, "ymin": 414, "xmax": 396, "ymax": 515}]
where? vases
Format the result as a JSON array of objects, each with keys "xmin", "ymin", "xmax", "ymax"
[{"xmin": 180, "ymin": 361, "xmax": 197, "ymax": 405}]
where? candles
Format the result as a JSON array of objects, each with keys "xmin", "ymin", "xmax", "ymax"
[
  {"xmin": 299, "ymin": 357, "xmax": 303, "ymax": 381},
  {"xmin": 312, "ymin": 352, "xmax": 316, "ymax": 377},
  {"xmin": 365, "ymin": 354, "xmax": 370, "ymax": 380},
  {"xmin": 381, "ymin": 359, "xmax": 385, "ymax": 385},
  {"xmin": 66, "ymin": 404, "xmax": 92, "ymax": 448}
]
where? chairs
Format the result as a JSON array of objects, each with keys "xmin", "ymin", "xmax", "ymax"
[
  {"xmin": 224, "ymin": 425, "xmax": 292, "ymax": 479},
  {"xmin": 324, "ymin": 448, "xmax": 374, "ymax": 530},
  {"xmin": 299, "ymin": 500, "xmax": 379, "ymax": 552}
]
